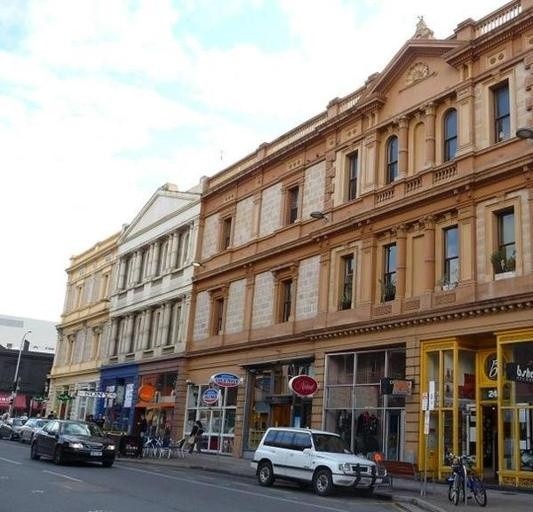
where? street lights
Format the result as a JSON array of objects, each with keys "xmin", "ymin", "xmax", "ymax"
[{"xmin": 8, "ymin": 330, "xmax": 31, "ymax": 415}]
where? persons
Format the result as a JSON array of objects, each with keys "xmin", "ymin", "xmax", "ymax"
[
  {"xmin": 95, "ymin": 413, "xmax": 104, "ymax": 428},
  {"xmin": 47, "ymin": 410, "xmax": 53, "ymax": 419},
  {"xmin": 87, "ymin": 414, "xmax": 95, "ymax": 422},
  {"xmin": 186, "ymin": 419, "xmax": 202, "ymax": 455}
]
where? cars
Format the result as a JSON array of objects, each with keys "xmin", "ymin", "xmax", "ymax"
[
  {"xmin": 30, "ymin": 419, "xmax": 117, "ymax": 468},
  {"xmin": 249, "ymin": 426, "xmax": 388, "ymax": 496},
  {"xmin": 0, "ymin": 413, "xmax": 44, "ymax": 443}
]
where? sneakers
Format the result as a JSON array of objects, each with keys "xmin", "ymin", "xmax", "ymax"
[{"xmin": 187, "ymin": 451, "xmax": 201, "ymax": 456}]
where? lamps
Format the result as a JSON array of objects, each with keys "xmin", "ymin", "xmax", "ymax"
[
  {"xmin": 515, "ymin": 127, "xmax": 533, "ymax": 141},
  {"xmin": 310, "ymin": 211, "xmax": 329, "ymax": 221},
  {"xmin": 190, "ymin": 261, "xmax": 204, "ymax": 269}
]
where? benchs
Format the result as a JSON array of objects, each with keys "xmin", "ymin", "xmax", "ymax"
[{"xmin": 382, "ymin": 460, "xmax": 422, "ymax": 493}]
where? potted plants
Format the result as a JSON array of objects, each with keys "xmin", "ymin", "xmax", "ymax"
[{"xmin": 490, "ymin": 251, "xmax": 506, "ymax": 275}]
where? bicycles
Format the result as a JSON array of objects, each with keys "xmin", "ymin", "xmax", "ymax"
[{"xmin": 447, "ymin": 452, "xmax": 486, "ymax": 507}]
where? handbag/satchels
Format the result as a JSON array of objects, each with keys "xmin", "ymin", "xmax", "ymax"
[{"xmin": 188, "ymin": 435, "xmax": 196, "ymax": 444}]
